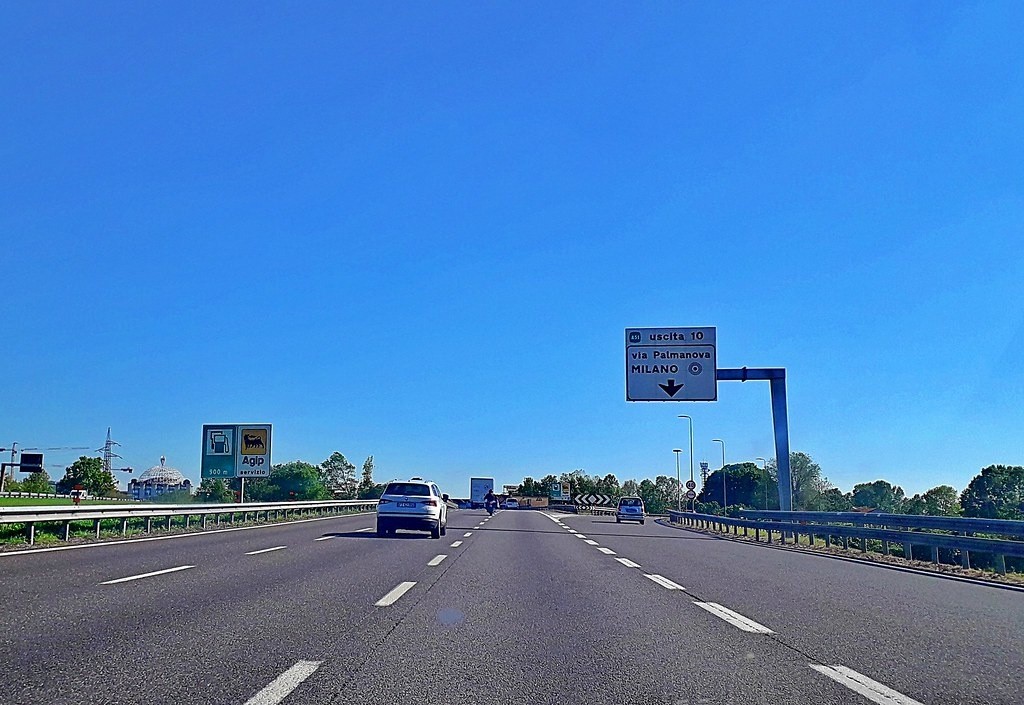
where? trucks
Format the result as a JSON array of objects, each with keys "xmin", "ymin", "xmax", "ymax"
[{"xmin": 470, "ymin": 477, "xmax": 494, "ymax": 509}]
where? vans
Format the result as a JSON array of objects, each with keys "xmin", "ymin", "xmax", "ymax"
[
  {"xmin": 70, "ymin": 490, "xmax": 87, "ymax": 500},
  {"xmin": 505, "ymin": 498, "xmax": 519, "ymax": 508}
]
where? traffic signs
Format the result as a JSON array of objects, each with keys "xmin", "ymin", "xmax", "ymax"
[{"xmin": 625, "ymin": 325, "xmax": 718, "ymax": 403}]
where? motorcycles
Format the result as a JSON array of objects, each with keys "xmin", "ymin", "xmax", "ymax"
[{"xmin": 485, "ymin": 494, "xmax": 496, "ymax": 514}]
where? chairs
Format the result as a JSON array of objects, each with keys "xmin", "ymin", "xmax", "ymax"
[
  {"xmin": 624, "ymin": 501, "xmax": 628, "ymax": 505},
  {"xmin": 397, "ymin": 487, "xmax": 405, "ymax": 493}
]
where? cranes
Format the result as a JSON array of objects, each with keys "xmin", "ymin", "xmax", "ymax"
[{"xmin": 0, "ymin": 441, "xmax": 90, "ymax": 478}]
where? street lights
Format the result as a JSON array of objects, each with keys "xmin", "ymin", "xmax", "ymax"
[
  {"xmin": 678, "ymin": 415, "xmax": 695, "ymax": 513},
  {"xmin": 757, "ymin": 458, "xmax": 768, "ymax": 512},
  {"xmin": 711, "ymin": 439, "xmax": 727, "ymax": 517},
  {"xmin": 673, "ymin": 449, "xmax": 682, "ymax": 511}
]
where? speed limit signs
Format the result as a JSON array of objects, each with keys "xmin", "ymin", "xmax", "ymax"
[{"xmin": 686, "ymin": 481, "xmax": 696, "ymax": 490}]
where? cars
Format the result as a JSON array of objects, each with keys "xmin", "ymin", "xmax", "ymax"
[{"xmin": 616, "ymin": 497, "xmax": 645, "ymax": 524}]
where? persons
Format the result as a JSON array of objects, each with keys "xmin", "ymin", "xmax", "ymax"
[{"xmin": 484, "ymin": 489, "xmax": 495, "ymax": 508}]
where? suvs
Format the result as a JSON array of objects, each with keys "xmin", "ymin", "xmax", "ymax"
[{"xmin": 376, "ymin": 480, "xmax": 450, "ymax": 539}]
